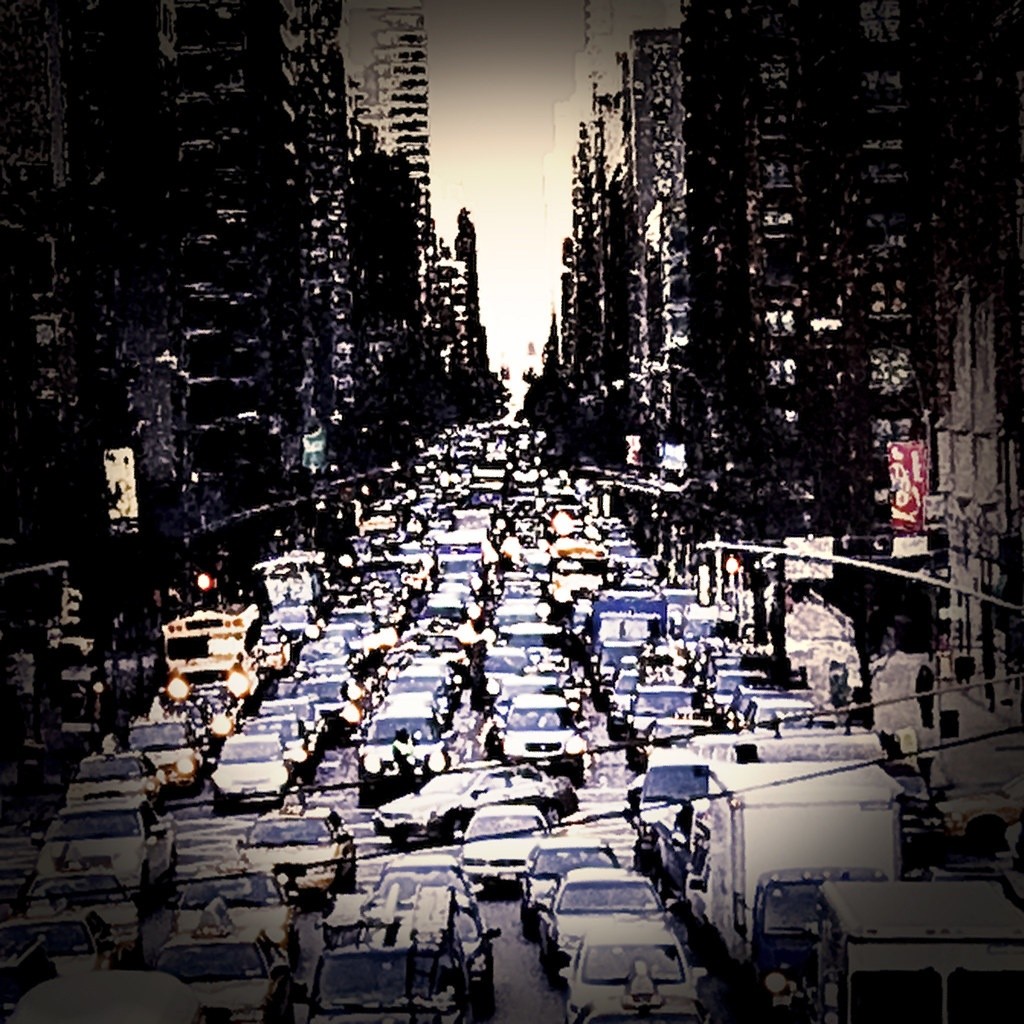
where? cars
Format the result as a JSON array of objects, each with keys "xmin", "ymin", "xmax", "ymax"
[{"xmin": 1, "ymin": 416, "xmax": 1024, "ymax": 1024}]
